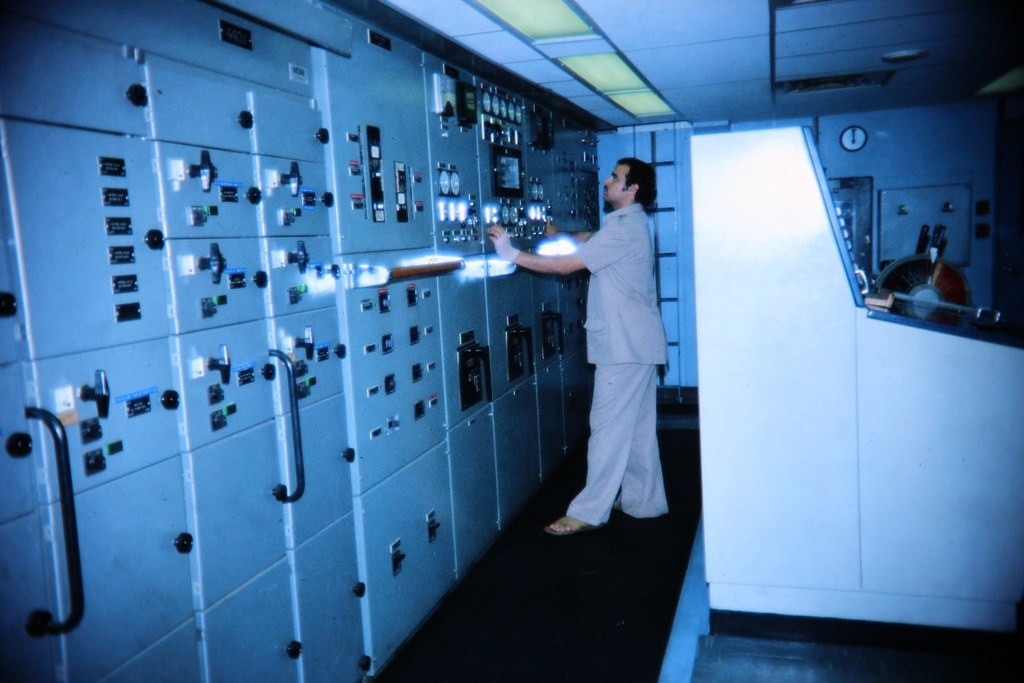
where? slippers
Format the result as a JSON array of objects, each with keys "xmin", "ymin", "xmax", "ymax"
[{"xmin": 544, "ymin": 516, "xmax": 592, "ymax": 534}]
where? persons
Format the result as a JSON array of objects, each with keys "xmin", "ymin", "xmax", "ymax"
[{"xmin": 488, "ymin": 158, "xmax": 667, "ymax": 537}]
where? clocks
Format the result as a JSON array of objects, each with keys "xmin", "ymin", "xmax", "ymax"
[{"xmin": 839, "ymin": 125, "xmax": 868, "ymax": 152}]
